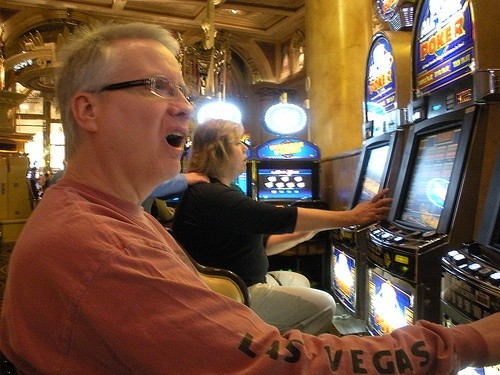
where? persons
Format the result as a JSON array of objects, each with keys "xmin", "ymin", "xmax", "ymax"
[
  {"xmin": 172, "ymin": 120, "xmax": 393, "ymax": 335},
  {"xmin": 0, "ymin": 22, "xmax": 500, "ymax": 375},
  {"xmin": 147, "ymin": 173, "xmax": 210, "ymax": 198}
]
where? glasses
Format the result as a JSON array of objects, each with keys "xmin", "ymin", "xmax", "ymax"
[{"xmin": 85, "ymin": 74, "xmax": 191, "ymax": 100}]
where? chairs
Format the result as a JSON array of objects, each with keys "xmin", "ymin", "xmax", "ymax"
[{"xmin": 164, "ymin": 227, "xmax": 251, "ymax": 306}]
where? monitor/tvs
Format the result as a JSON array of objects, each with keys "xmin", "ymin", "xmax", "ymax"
[
  {"xmin": 245, "ymin": 159, "xmax": 320, "ymax": 203},
  {"xmin": 325, "ymin": 236, "xmax": 358, "ymax": 317},
  {"xmin": 345, "ymin": 129, "xmax": 397, "ymax": 211},
  {"xmin": 387, "ymin": 102, "xmax": 479, "ymax": 237},
  {"xmin": 361, "ymin": 261, "xmax": 420, "ymax": 341}
]
